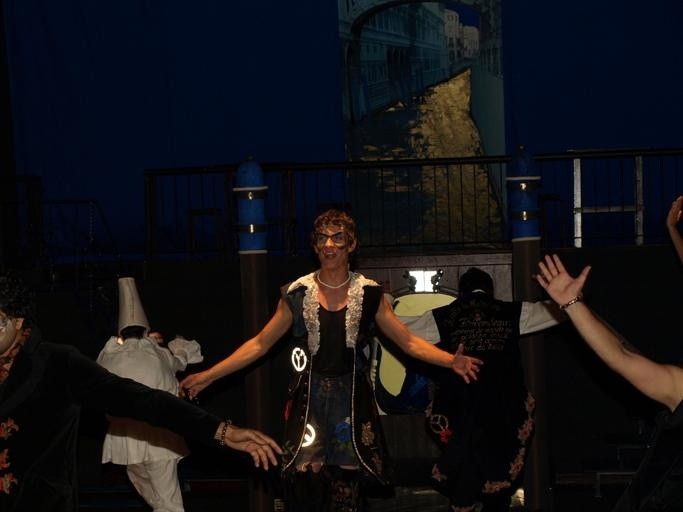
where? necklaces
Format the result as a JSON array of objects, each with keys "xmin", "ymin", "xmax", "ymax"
[{"xmin": 316, "ymin": 271, "xmax": 351, "ymax": 289}]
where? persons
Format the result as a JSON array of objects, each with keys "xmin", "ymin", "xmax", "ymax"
[
  {"xmin": 535, "ymin": 195, "xmax": 682, "ymax": 512},
  {"xmin": 148, "ymin": 328, "xmax": 165, "ymax": 347},
  {"xmin": 382, "ymin": 269, "xmax": 570, "ymax": 511},
  {"xmin": 0, "ymin": 272, "xmax": 285, "ymax": 512},
  {"xmin": 178, "ymin": 209, "xmax": 484, "ymax": 512},
  {"xmin": 96, "ymin": 277, "xmax": 205, "ymax": 512}
]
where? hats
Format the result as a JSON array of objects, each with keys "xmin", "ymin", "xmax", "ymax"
[{"xmin": 117, "ymin": 277, "xmax": 150, "ymax": 337}]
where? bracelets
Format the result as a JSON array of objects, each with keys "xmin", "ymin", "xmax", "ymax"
[
  {"xmin": 559, "ymin": 293, "xmax": 584, "ymax": 311},
  {"xmin": 220, "ymin": 417, "xmax": 232, "ymax": 446}
]
